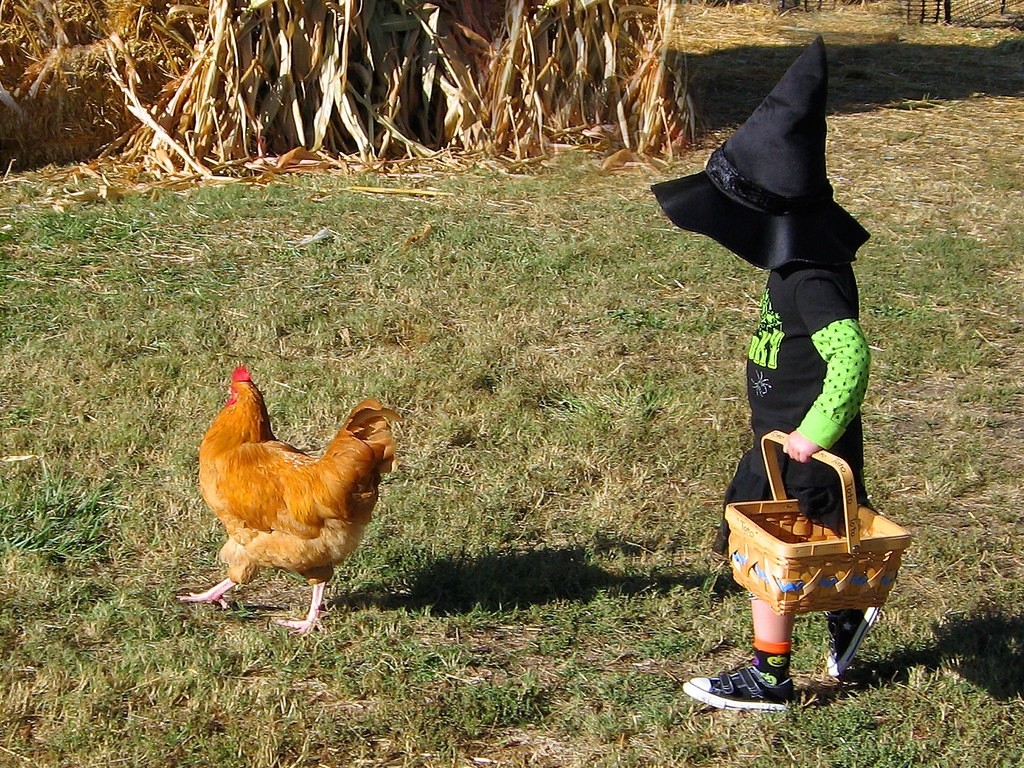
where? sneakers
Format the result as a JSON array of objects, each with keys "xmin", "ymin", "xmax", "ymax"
[
  {"xmin": 683, "ymin": 665, "xmax": 797, "ymax": 712},
  {"xmin": 826, "ymin": 602, "xmax": 879, "ymax": 677}
]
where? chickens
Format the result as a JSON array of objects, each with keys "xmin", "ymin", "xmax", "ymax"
[{"xmin": 175, "ymin": 360, "xmax": 404, "ymax": 634}]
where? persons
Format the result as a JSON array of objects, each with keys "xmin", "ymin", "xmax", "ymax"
[{"xmin": 650, "ymin": 36, "xmax": 880, "ymax": 713}]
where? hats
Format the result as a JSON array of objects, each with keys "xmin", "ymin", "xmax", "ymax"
[{"xmin": 650, "ymin": 33, "xmax": 874, "ymax": 270}]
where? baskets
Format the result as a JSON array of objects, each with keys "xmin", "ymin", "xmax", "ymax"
[{"xmin": 722, "ymin": 430, "xmax": 912, "ymax": 613}]
